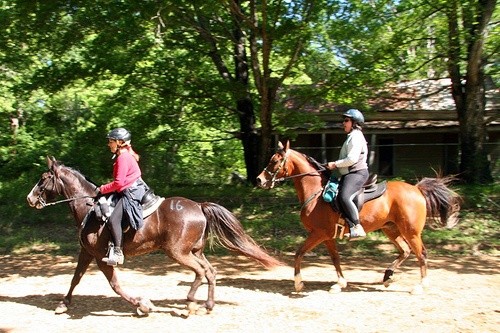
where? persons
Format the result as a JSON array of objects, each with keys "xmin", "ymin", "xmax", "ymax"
[
  {"xmin": 96, "ymin": 128, "xmax": 147, "ymax": 265},
  {"xmin": 325, "ymin": 109, "xmax": 370, "ymax": 240}
]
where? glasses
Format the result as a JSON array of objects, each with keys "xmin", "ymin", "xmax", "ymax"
[{"xmin": 344, "ymin": 119, "xmax": 351, "ymax": 123}]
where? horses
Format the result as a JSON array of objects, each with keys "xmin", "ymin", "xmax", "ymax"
[
  {"xmin": 256, "ymin": 140, "xmax": 463, "ymax": 292},
  {"xmin": 26, "ymin": 155, "xmax": 284, "ymax": 317}
]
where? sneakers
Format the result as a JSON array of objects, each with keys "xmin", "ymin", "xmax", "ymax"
[
  {"xmin": 344, "ymin": 223, "xmax": 366, "ymax": 238},
  {"xmin": 102, "ymin": 247, "xmax": 125, "ymax": 265}
]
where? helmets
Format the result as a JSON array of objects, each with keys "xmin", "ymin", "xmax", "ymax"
[
  {"xmin": 106, "ymin": 128, "xmax": 132, "ymax": 146},
  {"xmin": 341, "ymin": 109, "xmax": 364, "ymax": 126}
]
